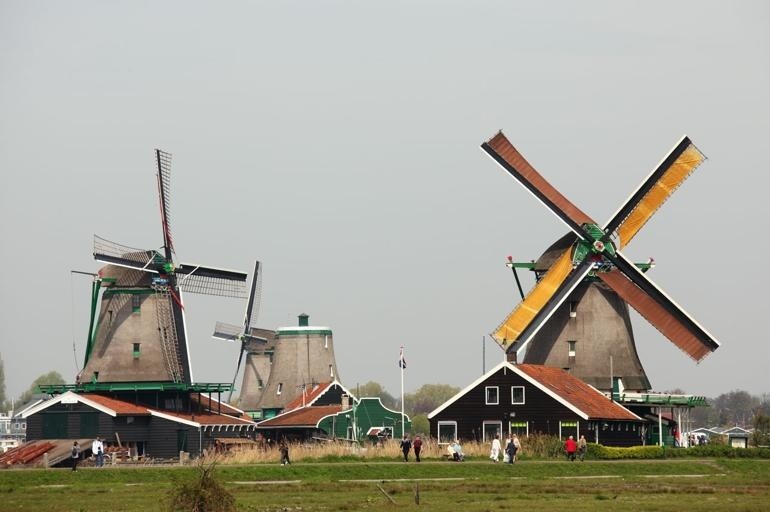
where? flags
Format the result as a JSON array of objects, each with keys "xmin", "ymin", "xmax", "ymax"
[{"xmin": 398, "ymin": 345, "xmax": 407, "ymax": 369}]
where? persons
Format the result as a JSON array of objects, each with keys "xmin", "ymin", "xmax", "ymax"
[
  {"xmin": 578, "ymin": 434, "xmax": 586, "ymax": 461},
  {"xmin": 70, "ymin": 440, "xmax": 80, "ymax": 473},
  {"xmin": 399, "ymin": 435, "xmax": 411, "ymax": 462},
  {"xmin": 566, "ymin": 435, "xmax": 577, "ymax": 463},
  {"xmin": 278, "ymin": 441, "xmax": 291, "ymax": 466},
  {"xmin": 672, "ymin": 426, "xmax": 708, "ymax": 448},
  {"xmin": 446, "ymin": 437, "xmax": 465, "ymax": 461},
  {"xmin": 92, "ymin": 434, "xmax": 105, "ymax": 468},
  {"xmin": 411, "ymin": 435, "xmax": 422, "ymax": 462},
  {"xmin": 489, "ymin": 433, "xmax": 522, "ymax": 465}
]
